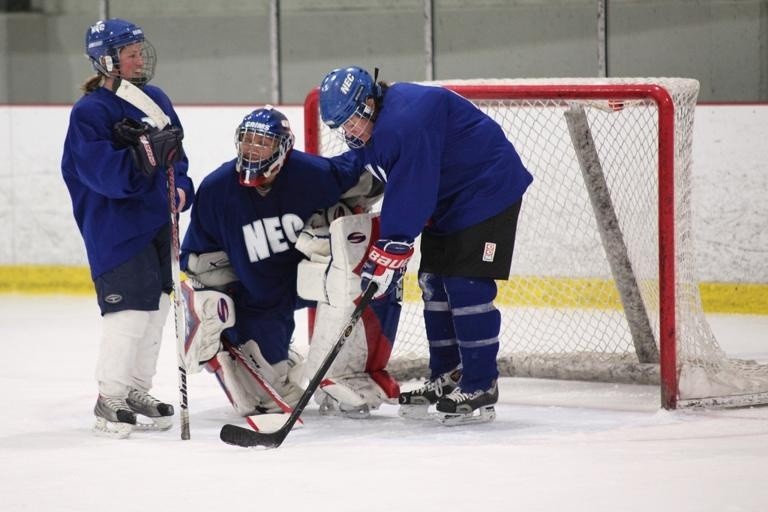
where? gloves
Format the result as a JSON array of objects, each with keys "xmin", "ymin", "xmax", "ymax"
[
  {"xmin": 113, "ymin": 116, "xmax": 184, "ymax": 179},
  {"xmin": 361, "ymin": 239, "xmax": 414, "ymax": 300}
]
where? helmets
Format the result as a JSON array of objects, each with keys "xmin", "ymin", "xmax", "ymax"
[
  {"xmin": 85, "ymin": 18, "xmax": 156, "ymax": 85},
  {"xmin": 234, "ymin": 105, "xmax": 295, "ymax": 187},
  {"xmin": 319, "ymin": 66, "xmax": 382, "ymax": 151}
]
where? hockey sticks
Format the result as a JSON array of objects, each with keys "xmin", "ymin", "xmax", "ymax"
[
  {"xmin": 112, "ymin": 76, "xmax": 190, "ymax": 440},
  {"xmin": 220, "ymin": 282, "xmax": 379, "ymax": 448}
]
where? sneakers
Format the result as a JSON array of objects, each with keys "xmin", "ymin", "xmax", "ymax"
[
  {"xmin": 95, "ymin": 394, "xmax": 136, "ymax": 424},
  {"xmin": 128, "ymin": 390, "xmax": 174, "ymax": 417},
  {"xmin": 424, "ymin": 364, "xmax": 464, "ymax": 405},
  {"xmin": 436, "ymin": 380, "xmax": 498, "ymax": 413}
]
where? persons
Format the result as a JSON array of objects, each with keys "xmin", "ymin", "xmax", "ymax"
[
  {"xmin": 318, "ymin": 66, "xmax": 535, "ymax": 428},
  {"xmin": 61, "ymin": 15, "xmax": 195, "ymax": 439},
  {"xmin": 172, "ymin": 103, "xmax": 409, "ymax": 420}
]
